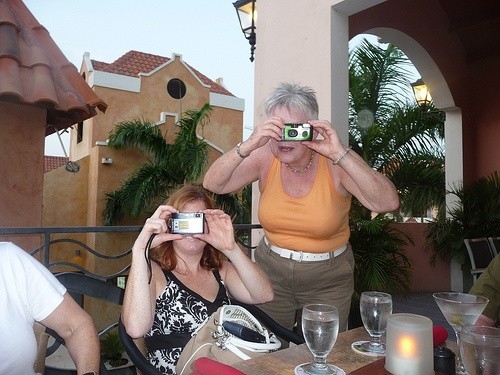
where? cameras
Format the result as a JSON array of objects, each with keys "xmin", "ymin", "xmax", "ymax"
[
  {"xmin": 170, "ymin": 212, "xmax": 204, "ymax": 234},
  {"xmin": 278, "ymin": 123, "xmax": 312, "ymax": 141}
]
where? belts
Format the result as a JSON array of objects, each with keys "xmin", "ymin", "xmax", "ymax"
[{"xmin": 263, "ymin": 236, "xmax": 347, "ymax": 262}]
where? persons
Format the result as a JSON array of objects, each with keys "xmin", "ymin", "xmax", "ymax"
[
  {"xmin": 203, "ymin": 82, "xmax": 400, "ymax": 353},
  {"xmin": 468, "ymin": 252, "xmax": 500, "ymax": 375},
  {"xmin": 121, "ymin": 183, "xmax": 275, "ymax": 375},
  {"xmin": 333, "ymin": 147, "xmax": 351, "ymax": 165},
  {"xmin": 0, "ymin": 242, "xmax": 100, "ymax": 375}
]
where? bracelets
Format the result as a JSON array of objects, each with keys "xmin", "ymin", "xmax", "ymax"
[{"xmin": 237, "ymin": 142, "xmax": 250, "ymax": 158}]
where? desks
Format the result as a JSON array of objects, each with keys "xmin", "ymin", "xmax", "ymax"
[{"xmin": 232, "ymin": 323, "xmax": 470, "ymax": 375}]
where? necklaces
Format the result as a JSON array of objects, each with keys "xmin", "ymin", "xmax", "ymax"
[{"xmin": 285, "ymin": 150, "xmax": 315, "ymax": 173}]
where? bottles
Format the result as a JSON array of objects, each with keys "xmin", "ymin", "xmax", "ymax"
[{"xmin": 434, "ymin": 345, "xmax": 455, "ymax": 375}]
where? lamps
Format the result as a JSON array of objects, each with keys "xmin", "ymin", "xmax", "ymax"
[
  {"xmin": 47, "ymin": 124, "xmax": 80, "ymax": 172},
  {"xmin": 411, "ymin": 78, "xmax": 432, "ymax": 113},
  {"xmin": 233, "ymin": 0, "xmax": 257, "ymax": 63}
]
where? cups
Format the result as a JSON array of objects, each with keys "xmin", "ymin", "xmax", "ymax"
[
  {"xmin": 459, "ymin": 325, "xmax": 500, "ymax": 375},
  {"xmin": 384, "ymin": 313, "xmax": 434, "ymax": 375}
]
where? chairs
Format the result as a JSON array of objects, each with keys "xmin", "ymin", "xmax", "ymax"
[
  {"xmin": 117, "ymin": 307, "xmax": 304, "ymax": 375},
  {"xmin": 463, "ymin": 237, "xmax": 500, "ymax": 283}
]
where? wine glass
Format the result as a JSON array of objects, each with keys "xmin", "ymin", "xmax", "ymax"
[
  {"xmin": 433, "ymin": 292, "xmax": 489, "ymax": 375},
  {"xmin": 351, "ymin": 292, "xmax": 393, "ymax": 356},
  {"xmin": 294, "ymin": 304, "xmax": 346, "ymax": 375}
]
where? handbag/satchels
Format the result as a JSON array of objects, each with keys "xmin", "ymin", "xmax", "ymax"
[{"xmin": 175, "ymin": 305, "xmax": 281, "ymax": 375}]
